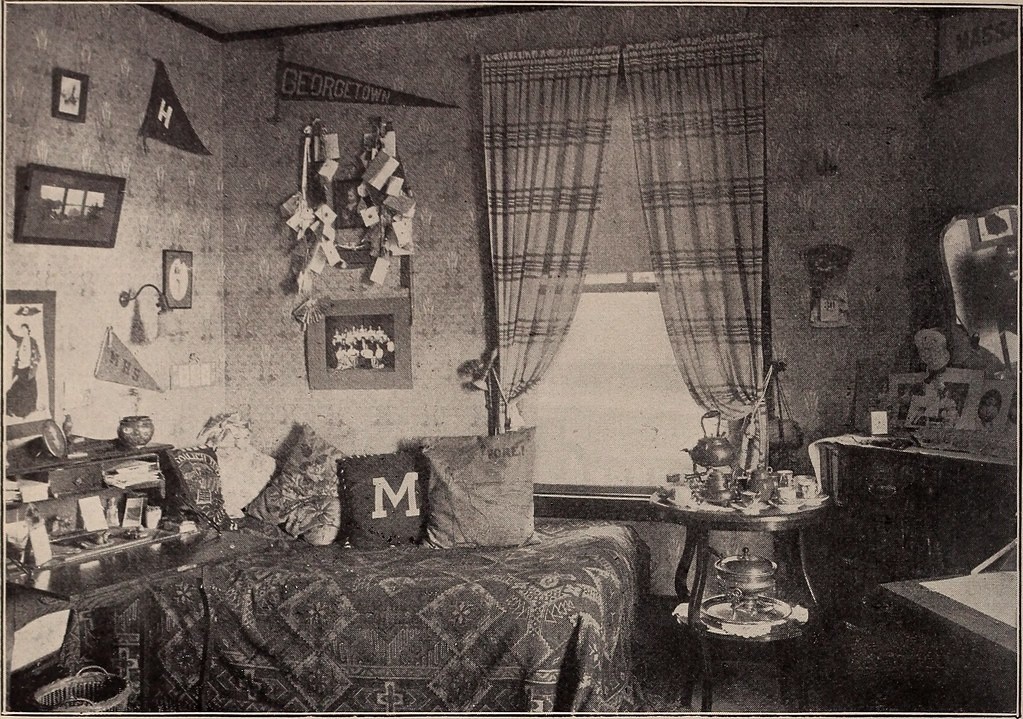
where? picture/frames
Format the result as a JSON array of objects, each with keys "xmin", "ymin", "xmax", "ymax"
[
  {"xmin": 13, "ymin": 163, "xmax": 126, "ymax": 250},
  {"xmin": 960, "ymin": 380, "xmax": 1015, "ymax": 432},
  {"xmin": 888, "ymin": 372, "xmax": 928, "ymax": 428},
  {"xmin": 300, "ymin": 162, "xmax": 378, "ymax": 268},
  {"xmin": 1, "ymin": 288, "xmax": 58, "ymax": 443},
  {"xmin": 904, "ymin": 393, "xmax": 956, "ymax": 429},
  {"xmin": 306, "ymin": 296, "xmax": 414, "ymax": 392},
  {"xmin": 161, "ymin": 248, "xmax": 194, "ymax": 311},
  {"xmin": 937, "ymin": 368, "xmax": 984, "ymax": 432},
  {"xmin": 51, "ymin": 67, "xmax": 89, "ymax": 124}
]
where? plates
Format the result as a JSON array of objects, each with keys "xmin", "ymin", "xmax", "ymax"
[
  {"xmin": 732, "ymin": 502, "xmax": 769, "ymax": 509},
  {"xmin": 769, "ymin": 499, "xmax": 804, "ymax": 509},
  {"xmin": 805, "ymin": 494, "xmax": 829, "ymax": 501}
]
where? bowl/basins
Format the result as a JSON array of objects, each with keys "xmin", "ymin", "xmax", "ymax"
[
  {"xmin": 118, "ymin": 416, "xmax": 154, "ymax": 446},
  {"xmin": 742, "ymin": 493, "xmax": 762, "ymax": 506},
  {"xmin": 714, "ymin": 546, "xmax": 777, "ymax": 593}
]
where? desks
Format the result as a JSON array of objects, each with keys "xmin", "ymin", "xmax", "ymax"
[
  {"xmin": 6, "ymin": 439, "xmax": 268, "ymax": 712},
  {"xmin": 650, "ymin": 490, "xmax": 833, "ymax": 712},
  {"xmin": 877, "ymin": 571, "xmax": 1017, "ymax": 666}
]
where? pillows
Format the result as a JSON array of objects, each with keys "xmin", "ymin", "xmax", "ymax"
[
  {"xmin": 418, "ymin": 424, "xmax": 542, "ymax": 553},
  {"xmin": 172, "ymin": 444, "xmax": 227, "ymax": 524},
  {"xmin": 215, "ymin": 427, "xmax": 276, "ymax": 521},
  {"xmin": 334, "ymin": 448, "xmax": 430, "ymax": 550},
  {"xmin": 237, "ymin": 421, "xmax": 348, "ymax": 548}
]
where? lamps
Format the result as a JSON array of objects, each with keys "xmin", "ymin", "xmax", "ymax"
[{"xmin": 119, "ymin": 283, "xmax": 185, "ymax": 346}]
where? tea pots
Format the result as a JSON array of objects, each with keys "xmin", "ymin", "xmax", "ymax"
[
  {"xmin": 682, "ymin": 410, "xmax": 735, "ymax": 466},
  {"xmin": 700, "ymin": 469, "xmax": 735, "ymax": 501}
]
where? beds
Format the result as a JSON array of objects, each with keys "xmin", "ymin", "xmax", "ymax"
[{"xmin": 57, "ymin": 517, "xmax": 654, "ymax": 712}]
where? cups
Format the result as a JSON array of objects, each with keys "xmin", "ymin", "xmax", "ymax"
[
  {"xmin": 778, "ymin": 488, "xmax": 792, "ymax": 502},
  {"xmin": 778, "ymin": 470, "xmax": 793, "ymax": 484},
  {"xmin": 795, "ymin": 475, "xmax": 816, "ymax": 497}
]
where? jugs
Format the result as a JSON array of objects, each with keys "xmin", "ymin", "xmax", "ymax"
[{"xmin": 748, "ymin": 464, "xmax": 774, "ymax": 498}]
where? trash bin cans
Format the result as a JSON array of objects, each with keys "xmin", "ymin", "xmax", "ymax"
[{"xmin": 33, "ymin": 664, "xmax": 130, "ymax": 713}]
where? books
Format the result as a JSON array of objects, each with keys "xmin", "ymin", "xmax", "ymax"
[{"xmin": 3, "ymin": 456, "xmax": 164, "ymax": 505}]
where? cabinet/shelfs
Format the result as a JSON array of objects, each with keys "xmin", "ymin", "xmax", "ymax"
[{"xmin": 800, "ymin": 431, "xmax": 1018, "ymax": 631}]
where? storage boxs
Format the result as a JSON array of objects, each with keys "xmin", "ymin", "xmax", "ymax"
[{"xmin": 15, "ymin": 471, "xmax": 51, "ymax": 504}]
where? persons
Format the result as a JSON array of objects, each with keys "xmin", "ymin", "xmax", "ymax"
[
  {"xmin": 171, "ymin": 263, "xmax": 185, "ymax": 300},
  {"xmin": 24, "ymin": 195, "xmax": 104, "ymax": 235},
  {"xmin": 327, "ymin": 322, "xmax": 395, "ymax": 370},
  {"xmin": 899, "ymin": 385, "xmax": 965, "ymax": 426}
]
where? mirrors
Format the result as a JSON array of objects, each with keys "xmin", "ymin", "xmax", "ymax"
[{"xmin": 937, "ymin": 205, "xmax": 1018, "ymax": 378}]
